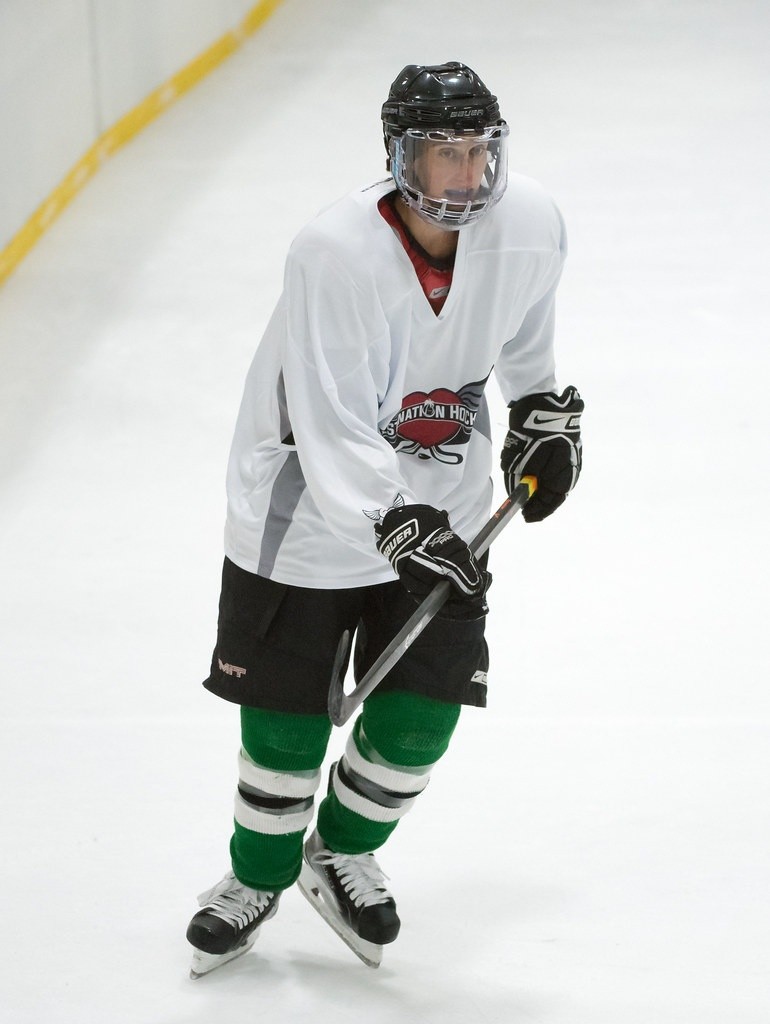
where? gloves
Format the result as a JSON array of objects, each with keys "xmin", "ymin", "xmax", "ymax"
[
  {"xmin": 498, "ymin": 383, "xmax": 584, "ymax": 525},
  {"xmin": 373, "ymin": 502, "xmax": 493, "ymax": 623}
]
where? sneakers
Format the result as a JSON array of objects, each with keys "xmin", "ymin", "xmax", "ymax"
[
  {"xmin": 185, "ymin": 870, "xmax": 284, "ymax": 980},
  {"xmin": 296, "ymin": 823, "xmax": 401, "ymax": 969}
]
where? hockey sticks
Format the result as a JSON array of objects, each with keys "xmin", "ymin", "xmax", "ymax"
[{"xmin": 326, "ymin": 470, "xmax": 538, "ymax": 729}]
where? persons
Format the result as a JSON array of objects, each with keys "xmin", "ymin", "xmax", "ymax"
[{"xmin": 185, "ymin": 60, "xmax": 585, "ymax": 983}]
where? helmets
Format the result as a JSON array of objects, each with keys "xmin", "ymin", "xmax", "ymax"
[{"xmin": 379, "ymin": 61, "xmax": 507, "ymax": 172}]
